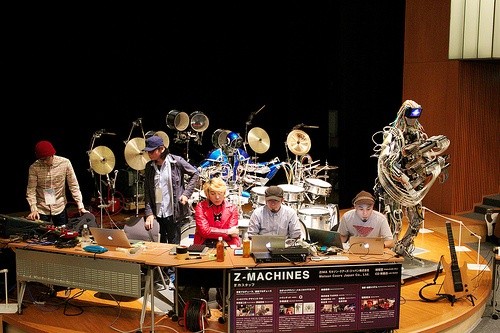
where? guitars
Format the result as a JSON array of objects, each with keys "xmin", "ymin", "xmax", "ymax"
[{"xmin": 443, "ymin": 221, "xmax": 472, "ymax": 298}]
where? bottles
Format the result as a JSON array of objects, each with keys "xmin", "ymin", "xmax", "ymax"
[
  {"xmin": 216, "ymin": 237, "xmax": 225, "ymax": 263},
  {"xmin": 242, "ymin": 232, "xmax": 250, "ymax": 259},
  {"xmin": 81, "ymin": 224, "xmax": 90, "ymax": 252}
]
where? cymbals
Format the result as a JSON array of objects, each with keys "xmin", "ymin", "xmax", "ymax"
[
  {"xmin": 315, "ymin": 166, "xmax": 340, "ymax": 170},
  {"xmin": 287, "ymin": 130, "xmax": 311, "ymax": 155},
  {"xmin": 244, "ymin": 175, "xmax": 267, "ymax": 185},
  {"xmin": 248, "ymin": 127, "xmax": 270, "ymax": 154},
  {"xmin": 89, "ymin": 146, "xmax": 115, "ymax": 175},
  {"xmin": 242, "ymin": 163, "xmax": 271, "ymax": 174},
  {"xmin": 124, "ymin": 137, "xmax": 151, "ymax": 170}
]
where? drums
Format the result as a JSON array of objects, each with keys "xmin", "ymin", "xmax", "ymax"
[
  {"xmin": 189, "ymin": 111, "xmax": 209, "ymax": 132},
  {"xmin": 200, "ymin": 190, "xmax": 229, "ymax": 202},
  {"xmin": 277, "ymin": 184, "xmax": 304, "ymax": 210},
  {"xmin": 212, "ymin": 129, "xmax": 232, "ymax": 149},
  {"xmin": 180, "ymin": 220, "xmax": 197, "ymax": 246},
  {"xmin": 145, "ymin": 130, "xmax": 170, "ymax": 149},
  {"xmin": 166, "ymin": 110, "xmax": 189, "ymax": 131},
  {"xmin": 303, "ymin": 179, "xmax": 333, "ymax": 196},
  {"xmin": 251, "ymin": 186, "xmax": 269, "ymax": 208},
  {"xmin": 297, "ymin": 208, "xmax": 332, "ymax": 241}
]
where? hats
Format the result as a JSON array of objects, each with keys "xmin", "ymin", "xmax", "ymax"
[
  {"xmin": 142, "ymin": 136, "xmax": 164, "ymax": 152},
  {"xmin": 352, "ymin": 191, "xmax": 375, "ymax": 204},
  {"xmin": 35, "ymin": 141, "xmax": 56, "ymax": 157},
  {"xmin": 264, "ymin": 186, "xmax": 284, "ymax": 200}
]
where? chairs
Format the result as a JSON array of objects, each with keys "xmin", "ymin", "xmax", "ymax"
[
  {"xmin": 70, "ymin": 211, "xmax": 97, "ymax": 236},
  {"xmin": 123, "ymin": 217, "xmax": 167, "ymax": 293}
]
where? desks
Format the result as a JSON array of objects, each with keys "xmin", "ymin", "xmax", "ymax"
[{"xmin": 0, "ymin": 232, "xmax": 405, "ymax": 333}]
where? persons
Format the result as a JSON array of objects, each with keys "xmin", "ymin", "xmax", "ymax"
[
  {"xmin": 26, "ymin": 140, "xmax": 89, "ymax": 293},
  {"xmin": 197, "ymin": 129, "xmax": 279, "ymax": 184},
  {"xmin": 246, "ymin": 186, "xmax": 302, "ymax": 240},
  {"xmin": 194, "ymin": 178, "xmax": 240, "ymax": 300},
  {"xmin": 279, "ymin": 306, "xmax": 294, "ymax": 315},
  {"xmin": 236, "ymin": 305, "xmax": 267, "ymax": 316},
  {"xmin": 361, "ymin": 299, "xmax": 393, "ymax": 311},
  {"xmin": 337, "ymin": 191, "xmax": 395, "ymax": 248},
  {"xmin": 141, "ymin": 136, "xmax": 199, "ymax": 290},
  {"xmin": 333, "ymin": 305, "xmax": 355, "ymax": 313}
]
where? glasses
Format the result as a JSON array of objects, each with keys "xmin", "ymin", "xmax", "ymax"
[{"xmin": 39, "ymin": 156, "xmax": 51, "ymax": 161}]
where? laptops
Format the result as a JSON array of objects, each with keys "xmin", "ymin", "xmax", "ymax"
[
  {"xmin": 251, "ymin": 236, "xmax": 285, "ymax": 254},
  {"xmin": 89, "ymin": 226, "xmax": 143, "ymax": 248},
  {"xmin": 307, "ymin": 228, "xmax": 350, "ymax": 251},
  {"xmin": 349, "ymin": 236, "xmax": 384, "ymax": 254}
]
